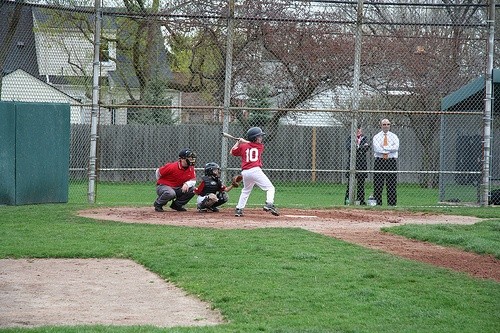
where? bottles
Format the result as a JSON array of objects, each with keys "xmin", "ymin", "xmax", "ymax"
[{"xmin": 369, "ymin": 194, "xmax": 373, "ymax": 198}]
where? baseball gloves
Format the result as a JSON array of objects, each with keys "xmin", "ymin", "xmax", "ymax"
[{"xmin": 233, "ymin": 175, "xmax": 243, "ymax": 187}]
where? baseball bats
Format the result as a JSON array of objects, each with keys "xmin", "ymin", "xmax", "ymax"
[{"xmin": 221, "ymin": 132, "xmax": 240, "ymax": 141}]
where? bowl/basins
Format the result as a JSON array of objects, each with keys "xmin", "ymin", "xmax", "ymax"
[
  {"xmin": 354, "ymin": 201, "xmax": 360, "ymax": 205},
  {"xmin": 368, "ymin": 200, "xmax": 376, "ymax": 206}
]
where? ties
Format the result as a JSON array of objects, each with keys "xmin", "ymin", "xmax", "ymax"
[{"xmin": 383, "ymin": 134, "xmax": 388, "ymax": 160}]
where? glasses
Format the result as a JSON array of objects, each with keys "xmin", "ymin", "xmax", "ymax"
[{"xmin": 383, "ymin": 124, "xmax": 391, "ymax": 126}]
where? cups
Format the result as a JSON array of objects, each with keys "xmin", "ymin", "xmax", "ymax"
[{"xmin": 346, "ymin": 200, "xmax": 349, "ymax": 205}]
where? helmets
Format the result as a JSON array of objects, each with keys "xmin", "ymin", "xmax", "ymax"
[
  {"xmin": 247, "ymin": 127, "xmax": 266, "ymax": 139},
  {"xmin": 205, "ymin": 163, "xmax": 220, "ymax": 170}
]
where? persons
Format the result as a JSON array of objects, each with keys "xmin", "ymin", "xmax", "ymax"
[
  {"xmin": 373, "ymin": 118, "xmax": 400, "ymax": 206},
  {"xmin": 345, "ymin": 122, "xmax": 370, "ymax": 206},
  {"xmin": 192, "ymin": 162, "xmax": 234, "ymax": 214},
  {"xmin": 153, "ymin": 149, "xmax": 199, "ymax": 212},
  {"xmin": 230, "ymin": 127, "xmax": 281, "ymax": 217}
]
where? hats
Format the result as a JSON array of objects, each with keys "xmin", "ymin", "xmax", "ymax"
[{"xmin": 179, "ymin": 149, "xmax": 186, "ymax": 157}]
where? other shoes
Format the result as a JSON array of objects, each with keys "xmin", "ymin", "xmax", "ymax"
[
  {"xmin": 154, "ymin": 202, "xmax": 164, "ymax": 212},
  {"xmin": 263, "ymin": 203, "xmax": 280, "ymax": 216},
  {"xmin": 169, "ymin": 205, "xmax": 187, "ymax": 211},
  {"xmin": 196, "ymin": 204, "xmax": 207, "ymax": 212},
  {"xmin": 209, "ymin": 206, "xmax": 219, "ymax": 212},
  {"xmin": 235, "ymin": 208, "xmax": 244, "ymax": 216}
]
